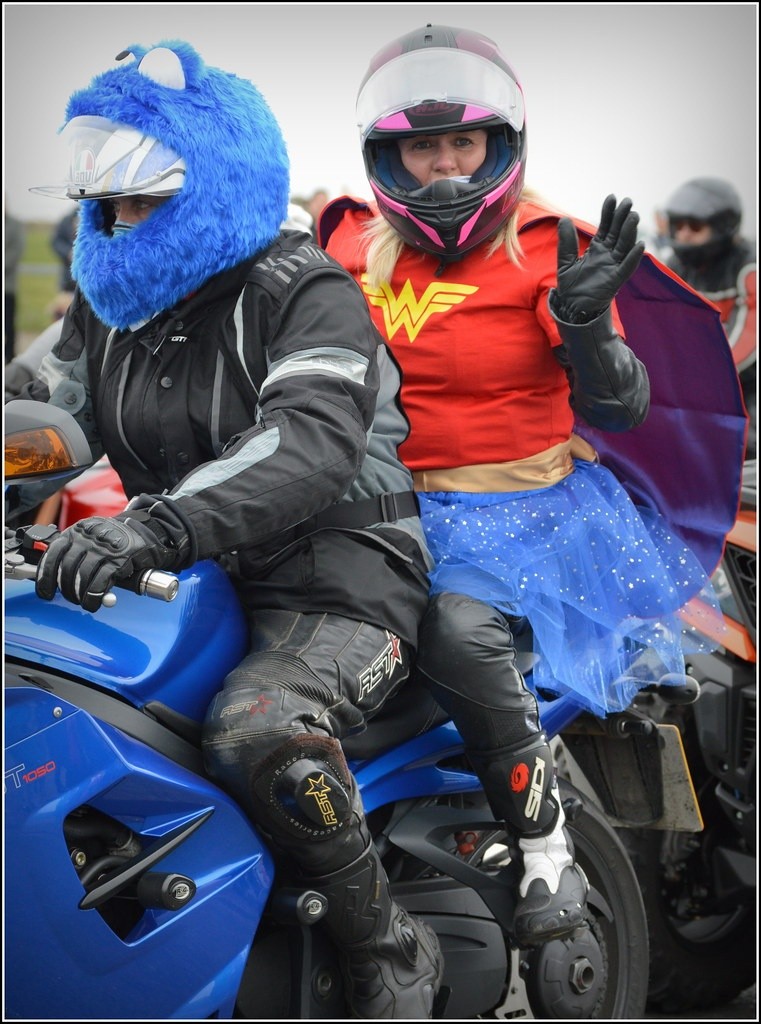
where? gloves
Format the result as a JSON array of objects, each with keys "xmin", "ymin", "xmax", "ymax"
[
  {"xmin": 551, "ymin": 195, "xmax": 644, "ymax": 321},
  {"xmin": 35, "ymin": 509, "xmax": 176, "ymax": 612}
]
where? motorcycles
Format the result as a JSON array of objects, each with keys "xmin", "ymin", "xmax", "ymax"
[
  {"xmin": 1, "ymin": 397, "xmax": 705, "ymax": 1021},
  {"xmin": 571, "ymin": 457, "xmax": 760, "ymax": 1011}
]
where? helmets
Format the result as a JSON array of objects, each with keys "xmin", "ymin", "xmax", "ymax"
[
  {"xmin": 663, "ymin": 179, "xmax": 744, "ymax": 271},
  {"xmin": 354, "ymin": 26, "xmax": 525, "ymax": 257},
  {"xmin": 30, "ymin": 40, "xmax": 289, "ymax": 330}
]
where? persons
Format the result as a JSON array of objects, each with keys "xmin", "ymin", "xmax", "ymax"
[
  {"xmin": 4, "ymin": 35, "xmax": 443, "ymax": 1017},
  {"xmin": 656, "ymin": 176, "xmax": 756, "ymax": 404},
  {"xmin": 316, "ymin": 28, "xmax": 651, "ymax": 943},
  {"xmin": 4, "ymin": 207, "xmax": 81, "ymax": 367},
  {"xmin": 306, "ymin": 189, "xmax": 329, "ymax": 246}
]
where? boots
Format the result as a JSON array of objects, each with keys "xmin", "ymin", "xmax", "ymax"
[
  {"xmin": 466, "ymin": 729, "xmax": 592, "ymax": 945},
  {"xmin": 304, "ymin": 829, "xmax": 445, "ymax": 1019}
]
